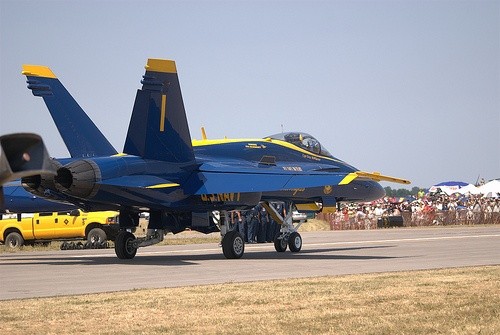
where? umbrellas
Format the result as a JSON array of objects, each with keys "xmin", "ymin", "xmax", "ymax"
[{"xmin": 428, "ymin": 181, "xmax": 476, "ymax": 193}]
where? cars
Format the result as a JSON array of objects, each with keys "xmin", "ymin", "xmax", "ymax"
[{"xmin": 293, "ymin": 210, "xmax": 308, "ymax": 222}]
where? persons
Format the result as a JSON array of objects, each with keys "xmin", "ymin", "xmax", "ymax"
[
  {"xmin": 330, "ymin": 189, "xmax": 500, "ymax": 228},
  {"xmin": 219, "ymin": 201, "xmax": 293, "ymax": 243}
]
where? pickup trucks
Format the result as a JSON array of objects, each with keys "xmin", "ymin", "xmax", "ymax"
[{"xmin": 1, "ymin": 209, "xmax": 120, "ymax": 248}]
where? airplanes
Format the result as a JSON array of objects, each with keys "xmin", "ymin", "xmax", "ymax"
[{"xmin": 0, "ymin": 58, "xmax": 411, "ymax": 259}]
